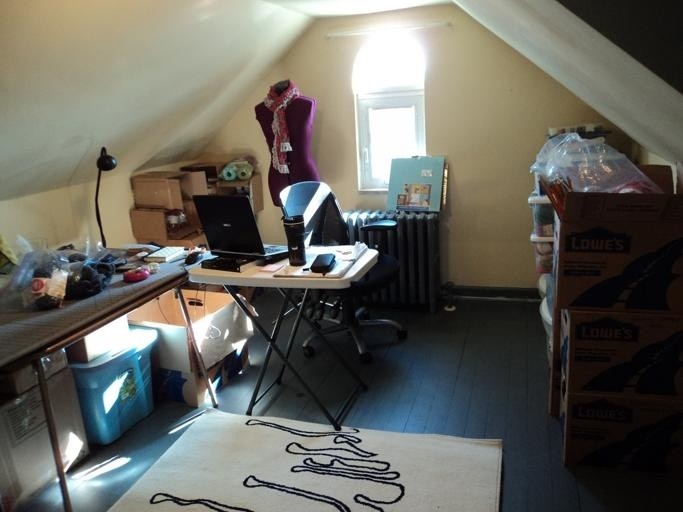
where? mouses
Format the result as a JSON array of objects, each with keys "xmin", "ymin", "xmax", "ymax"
[{"xmin": 185, "ymin": 252, "xmax": 202, "ymax": 263}]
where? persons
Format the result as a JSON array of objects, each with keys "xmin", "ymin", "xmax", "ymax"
[{"xmin": 253, "ymin": 80, "xmax": 321, "ymax": 206}]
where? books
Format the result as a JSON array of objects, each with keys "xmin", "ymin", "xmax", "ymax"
[
  {"xmin": 143, "ymin": 245, "xmax": 186, "ymax": 264},
  {"xmin": 201, "ymin": 255, "xmax": 258, "ymax": 273},
  {"xmin": 271, "ymin": 257, "xmax": 357, "ymax": 278}
]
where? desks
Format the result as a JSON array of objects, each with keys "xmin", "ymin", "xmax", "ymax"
[
  {"xmin": 189, "ymin": 246, "xmax": 381, "ymax": 431},
  {"xmin": 0, "ymin": 261, "xmax": 218, "ymax": 512}
]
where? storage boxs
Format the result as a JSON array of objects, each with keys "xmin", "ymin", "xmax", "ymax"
[
  {"xmin": 129, "ymin": 288, "xmax": 258, "ymax": 410},
  {"xmin": 130, "ymin": 171, "xmax": 262, "ymax": 250},
  {"xmin": 0, "ymin": 314, "xmax": 162, "ymax": 512}
]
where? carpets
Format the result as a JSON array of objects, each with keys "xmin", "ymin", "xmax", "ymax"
[{"xmin": 104, "ymin": 407, "xmax": 502, "ymax": 512}]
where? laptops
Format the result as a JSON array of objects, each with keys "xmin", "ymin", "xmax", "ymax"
[{"xmin": 192, "ymin": 194, "xmax": 289, "ymax": 264}]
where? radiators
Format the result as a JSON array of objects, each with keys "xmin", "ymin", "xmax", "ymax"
[{"xmin": 342, "ymin": 210, "xmax": 440, "ymax": 316}]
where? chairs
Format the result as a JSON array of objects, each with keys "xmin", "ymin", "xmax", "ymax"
[{"xmin": 279, "ymin": 181, "xmax": 406, "ymax": 361}]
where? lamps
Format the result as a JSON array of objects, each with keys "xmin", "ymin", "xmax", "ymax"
[{"xmin": 95, "ymin": 147, "xmax": 117, "ymax": 248}]
